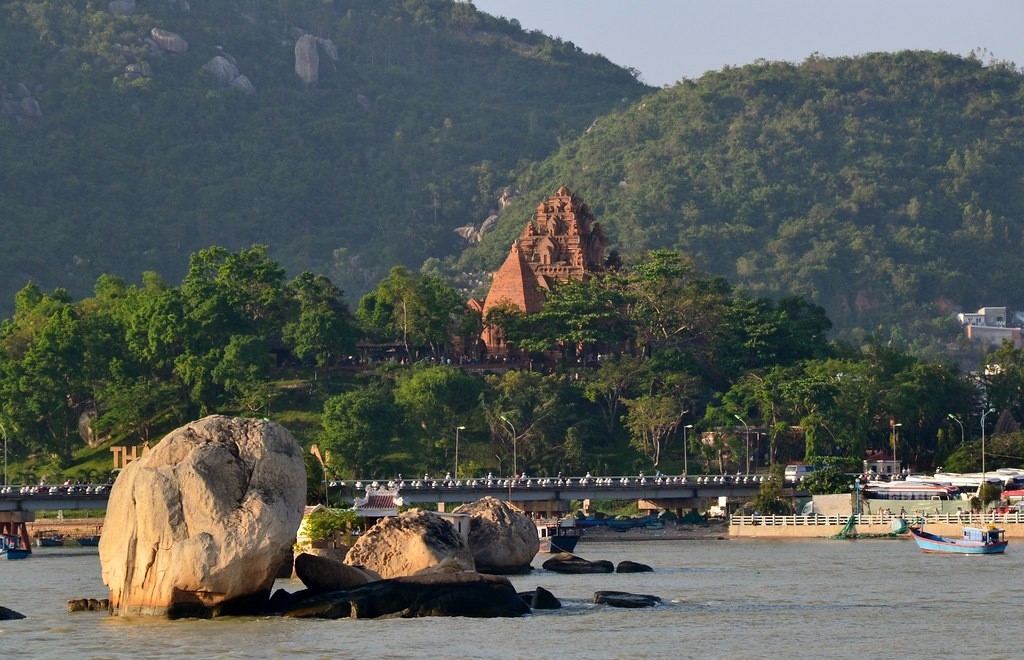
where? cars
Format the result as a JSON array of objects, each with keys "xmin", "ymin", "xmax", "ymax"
[{"xmin": 863, "ymin": 467, "xmax": 1024, "ymax": 499}]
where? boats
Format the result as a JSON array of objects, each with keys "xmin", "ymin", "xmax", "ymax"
[
  {"xmin": 537, "ymin": 535, "xmax": 580, "ymax": 554},
  {"xmin": 908, "ymin": 525, "xmax": 1009, "ymax": 554},
  {"xmin": 76, "ymin": 535, "xmax": 100, "ymax": 546},
  {"xmin": 37, "ymin": 537, "xmax": 65, "ymax": 546},
  {"xmin": 0, "ymin": 534, "xmax": 32, "ymax": 560},
  {"xmin": 575, "ymin": 515, "xmax": 665, "ymax": 532}
]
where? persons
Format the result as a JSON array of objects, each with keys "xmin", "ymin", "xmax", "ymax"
[
  {"xmin": 935, "ymin": 507, "xmax": 940, "ymax": 522},
  {"xmin": 753, "ymin": 508, "xmax": 761, "ymax": 525},
  {"xmin": 987, "ymin": 507, "xmax": 997, "ymax": 523},
  {"xmin": 327, "ymin": 352, "xmax": 605, "ymax": 382},
  {"xmin": 957, "ymin": 506, "xmax": 962, "ymax": 525},
  {"xmin": 526, "ymin": 512, "xmax": 558, "ymax": 521},
  {"xmin": 935, "ymin": 466, "xmax": 942, "ymax": 474},
  {"xmin": 1, "ymin": 465, "xmax": 912, "ymax": 496},
  {"xmin": 876, "ymin": 505, "xmax": 920, "ymax": 521}
]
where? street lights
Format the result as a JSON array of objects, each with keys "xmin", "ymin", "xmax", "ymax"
[
  {"xmin": 980, "ymin": 408, "xmax": 997, "ymax": 515},
  {"xmin": 684, "ymin": 424, "xmax": 693, "ymax": 475},
  {"xmin": 735, "ymin": 414, "xmax": 749, "ymax": 476},
  {"xmin": 455, "ymin": 426, "xmax": 466, "ymax": 484},
  {"xmin": 893, "ymin": 424, "xmax": 902, "ymax": 475},
  {"xmin": 948, "ymin": 414, "xmax": 964, "ymax": 446},
  {"xmin": 500, "ymin": 415, "xmax": 517, "ymax": 477}
]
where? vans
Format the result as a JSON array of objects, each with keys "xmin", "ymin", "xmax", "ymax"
[{"xmin": 784, "ymin": 465, "xmax": 820, "ymax": 484}]
